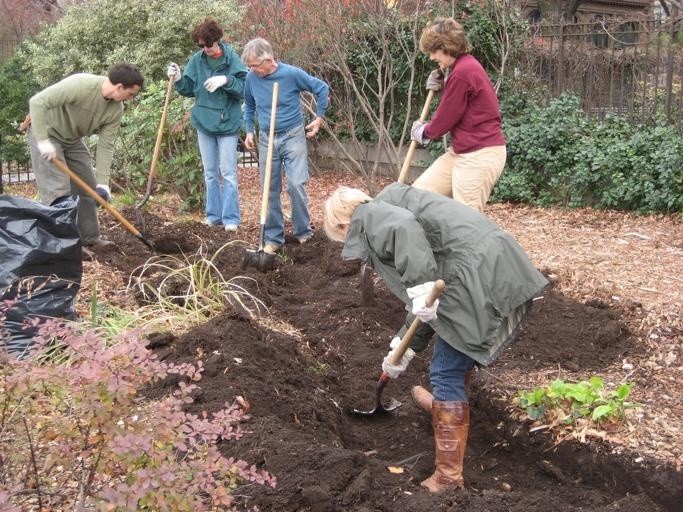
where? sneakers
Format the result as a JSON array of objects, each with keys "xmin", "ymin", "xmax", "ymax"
[
  {"xmin": 261, "ymin": 231, "xmax": 285, "ymax": 254},
  {"xmin": 80, "ymin": 238, "xmax": 116, "ymax": 261},
  {"xmin": 225, "ymin": 223, "xmax": 238, "ymax": 233},
  {"xmin": 202, "ymin": 218, "xmax": 221, "ymax": 229}
]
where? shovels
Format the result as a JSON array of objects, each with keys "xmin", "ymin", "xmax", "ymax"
[
  {"xmin": 358, "ymin": 69, "xmax": 444, "ymax": 306},
  {"xmin": 241, "ymin": 82, "xmax": 279, "ymax": 274},
  {"xmin": 134, "ymin": 65, "xmax": 175, "ymax": 237},
  {"xmin": 45, "ymin": 156, "xmax": 169, "ymax": 257},
  {"xmin": 350, "ymin": 279, "xmax": 445, "ymax": 415}
]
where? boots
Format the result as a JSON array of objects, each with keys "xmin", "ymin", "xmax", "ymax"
[
  {"xmin": 294, "ymin": 232, "xmax": 314, "ymax": 245},
  {"xmin": 409, "ymin": 385, "xmax": 471, "ymax": 495}
]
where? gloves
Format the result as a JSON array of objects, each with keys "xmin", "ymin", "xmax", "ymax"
[
  {"xmin": 409, "ymin": 120, "xmax": 432, "ymax": 146},
  {"xmin": 202, "ymin": 75, "xmax": 226, "ymax": 93},
  {"xmin": 166, "ymin": 62, "xmax": 181, "ymax": 83},
  {"xmin": 425, "ymin": 68, "xmax": 446, "ymax": 92},
  {"xmin": 35, "ymin": 139, "xmax": 57, "ymax": 162},
  {"xmin": 89, "ymin": 183, "xmax": 110, "ymax": 209},
  {"xmin": 406, "ymin": 280, "xmax": 442, "ymax": 324},
  {"xmin": 379, "ymin": 336, "xmax": 417, "ymax": 380}
]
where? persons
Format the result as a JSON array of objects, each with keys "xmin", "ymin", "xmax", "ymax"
[
  {"xmin": 241, "ymin": 38, "xmax": 328, "ymax": 251},
  {"xmin": 410, "ymin": 18, "xmax": 507, "ymax": 214},
  {"xmin": 25, "ymin": 65, "xmax": 143, "ymax": 260},
  {"xmin": 166, "ymin": 18, "xmax": 250, "ymax": 231},
  {"xmin": 323, "ymin": 181, "xmax": 550, "ymax": 492}
]
